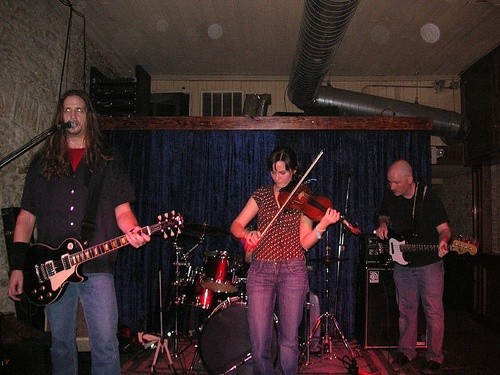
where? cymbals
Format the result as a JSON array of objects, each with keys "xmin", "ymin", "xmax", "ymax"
[
  {"xmin": 310, "ymin": 256, "xmax": 343, "ymax": 264},
  {"xmin": 183, "ymin": 223, "xmax": 227, "ymax": 236}
]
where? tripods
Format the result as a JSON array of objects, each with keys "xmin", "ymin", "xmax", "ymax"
[
  {"xmin": 298, "ymin": 260, "xmax": 358, "ymax": 366},
  {"xmin": 131, "ymin": 233, "xmax": 192, "ymax": 375}
]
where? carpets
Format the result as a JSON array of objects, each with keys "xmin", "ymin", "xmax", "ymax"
[{"xmin": 121, "ymin": 341, "xmax": 405, "ymax": 375}]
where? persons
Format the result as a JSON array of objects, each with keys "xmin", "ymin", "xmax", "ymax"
[
  {"xmin": 309, "ymin": 292, "xmax": 322, "ymax": 355},
  {"xmin": 230, "ymin": 147, "xmax": 340, "ymax": 375},
  {"xmin": 376, "ymin": 159, "xmax": 452, "ymax": 375},
  {"xmin": 9, "ymin": 90, "xmax": 150, "ymax": 375}
]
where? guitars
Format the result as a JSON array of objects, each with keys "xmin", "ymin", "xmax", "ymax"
[
  {"xmin": 22, "ymin": 211, "xmax": 186, "ymax": 306},
  {"xmin": 356, "ymin": 230, "xmax": 480, "ymax": 267}
]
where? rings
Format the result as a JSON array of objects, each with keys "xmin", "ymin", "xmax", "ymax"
[
  {"xmin": 251, "ymin": 233, "xmax": 253, "ymax": 236},
  {"xmin": 143, "ymin": 241, "xmax": 145, "ymax": 244}
]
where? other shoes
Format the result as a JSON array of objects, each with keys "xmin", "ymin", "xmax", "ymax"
[
  {"xmin": 430, "ymin": 359, "xmax": 441, "ymax": 372},
  {"xmin": 390, "ymin": 352, "xmax": 411, "ymax": 372}
]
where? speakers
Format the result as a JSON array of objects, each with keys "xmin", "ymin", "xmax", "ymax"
[
  {"xmin": 44, "ymin": 302, "xmax": 90, "ymax": 353},
  {"xmin": 354, "ymin": 265, "xmax": 429, "ymax": 351}
]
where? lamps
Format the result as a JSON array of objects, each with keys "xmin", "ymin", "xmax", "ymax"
[{"xmin": 430, "ymin": 145, "xmax": 444, "ymax": 164}]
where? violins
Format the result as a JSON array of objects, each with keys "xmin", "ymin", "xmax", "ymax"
[{"xmin": 277, "ymin": 182, "xmax": 362, "ymax": 235}]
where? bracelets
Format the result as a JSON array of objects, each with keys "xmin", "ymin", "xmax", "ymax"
[{"xmin": 316, "ymin": 225, "xmax": 326, "ymax": 232}]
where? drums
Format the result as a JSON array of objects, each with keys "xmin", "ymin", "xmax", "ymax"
[
  {"xmin": 188, "ymin": 266, "xmax": 214, "ymax": 310},
  {"xmin": 197, "ymin": 296, "xmax": 280, "ymax": 375},
  {"xmin": 201, "ymin": 250, "xmax": 240, "ymax": 293},
  {"xmin": 233, "ymin": 260, "xmax": 250, "ymax": 282}
]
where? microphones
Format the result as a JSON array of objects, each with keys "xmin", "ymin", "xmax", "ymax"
[{"xmin": 54, "ymin": 119, "xmax": 76, "ymax": 129}]
